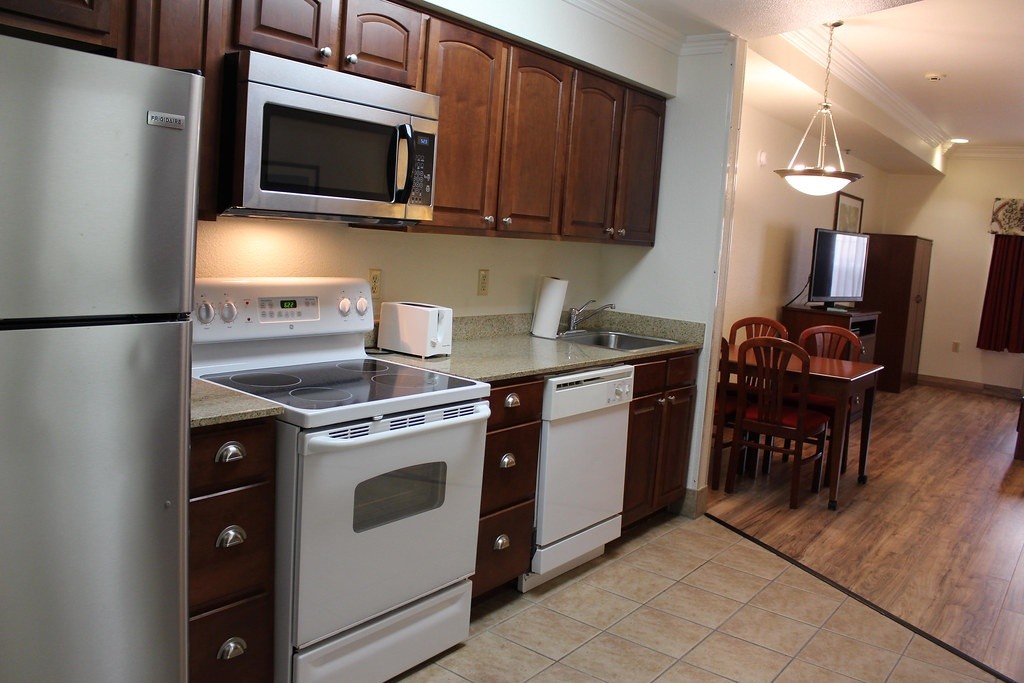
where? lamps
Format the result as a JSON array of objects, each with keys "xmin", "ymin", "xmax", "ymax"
[{"xmin": 774, "ymin": 21, "xmax": 865, "ymax": 196}]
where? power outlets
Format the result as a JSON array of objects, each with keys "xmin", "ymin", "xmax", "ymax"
[
  {"xmin": 368, "ymin": 267, "xmax": 381, "ymax": 299},
  {"xmin": 478, "ymin": 270, "xmax": 489, "ymax": 295}
]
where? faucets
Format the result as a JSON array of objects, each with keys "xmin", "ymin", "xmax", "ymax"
[{"xmin": 569, "ymin": 299, "xmax": 616, "ymax": 330}]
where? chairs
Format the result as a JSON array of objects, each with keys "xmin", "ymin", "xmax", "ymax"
[
  {"xmin": 725, "ymin": 338, "xmax": 831, "ymax": 508},
  {"xmin": 784, "ymin": 326, "xmax": 862, "ymax": 474},
  {"xmin": 721, "ymin": 317, "xmax": 790, "ymax": 392},
  {"xmin": 709, "ymin": 337, "xmax": 747, "ymax": 489}
]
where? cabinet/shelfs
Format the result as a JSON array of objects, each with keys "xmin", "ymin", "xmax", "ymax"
[
  {"xmin": 862, "ymin": 232, "xmax": 933, "ymax": 393},
  {"xmin": 623, "ymin": 354, "xmax": 698, "ymax": 526},
  {"xmin": 187, "ymin": 419, "xmax": 273, "ymax": 683},
  {"xmin": 473, "ymin": 380, "xmax": 543, "ymax": 594},
  {"xmin": 421, "ymin": 18, "xmax": 572, "ymax": 236},
  {"xmin": 561, "ymin": 69, "xmax": 667, "ymax": 246},
  {"xmin": 233, "ymin": 0, "xmax": 425, "ymax": 83}
]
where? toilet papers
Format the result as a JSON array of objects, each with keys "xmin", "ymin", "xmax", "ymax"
[{"xmin": 530, "ymin": 273, "xmax": 569, "ymax": 339}]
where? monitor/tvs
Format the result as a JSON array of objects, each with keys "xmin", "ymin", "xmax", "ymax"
[{"xmin": 808, "ymin": 227, "xmax": 870, "ymax": 311}]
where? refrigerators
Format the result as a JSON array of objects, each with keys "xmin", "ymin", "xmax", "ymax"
[{"xmin": 1, "ymin": 32, "xmax": 206, "ymax": 683}]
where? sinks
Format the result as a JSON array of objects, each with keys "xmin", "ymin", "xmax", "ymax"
[{"xmin": 558, "ymin": 330, "xmax": 680, "ymax": 352}]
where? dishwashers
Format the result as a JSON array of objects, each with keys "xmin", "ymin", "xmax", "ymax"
[{"xmin": 532, "ymin": 364, "xmax": 635, "ymax": 576}]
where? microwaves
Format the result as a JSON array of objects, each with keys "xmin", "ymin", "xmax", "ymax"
[{"xmin": 215, "ymin": 48, "xmax": 440, "ymax": 229}]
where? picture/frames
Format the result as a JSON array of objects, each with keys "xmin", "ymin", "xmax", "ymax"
[{"xmin": 834, "ymin": 189, "xmax": 864, "ymax": 233}]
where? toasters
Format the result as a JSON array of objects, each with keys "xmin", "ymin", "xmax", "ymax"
[{"xmin": 377, "ymin": 301, "xmax": 453, "ymax": 360}]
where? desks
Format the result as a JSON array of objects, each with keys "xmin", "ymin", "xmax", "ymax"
[{"xmin": 718, "ymin": 344, "xmax": 884, "ymax": 509}]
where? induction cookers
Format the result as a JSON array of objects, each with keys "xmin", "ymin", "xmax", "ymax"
[{"xmin": 192, "ymin": 275, "xmax": 491, "ymax": 428}]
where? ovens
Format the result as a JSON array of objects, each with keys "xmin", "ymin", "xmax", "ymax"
[{"xmin": 275, "ymin": 400, "xmax": 491, "ymax": 682}]
